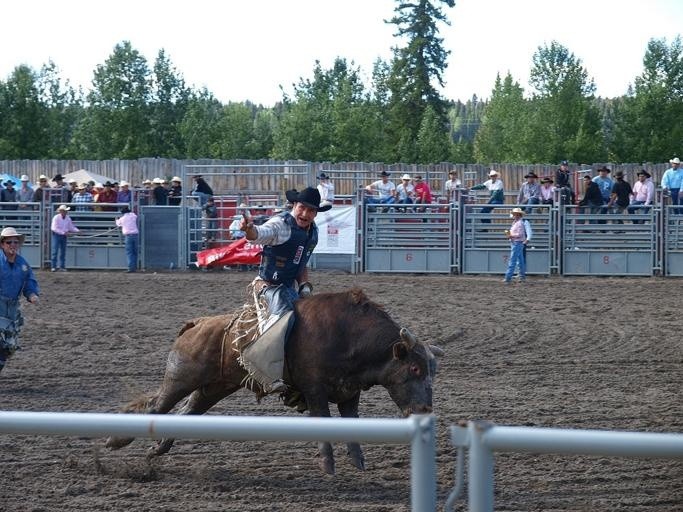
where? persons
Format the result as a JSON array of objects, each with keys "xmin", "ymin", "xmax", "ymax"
[
  {"xmin": 517, "ymin": 161, "xmax": 576, "ymax": 219},
  {"xmin": 0, "ymin": 175, "xmax": 183, "ymax": 229},
  {"xmin": 51, "ymin": 204, "xmax": 80, "ymax": 271},
  {"xmin": 367, "ymin": 171, "xmax": 432, "ymax": 213},
  {"xmin": 0, "ymin": 227, "xmax": 40, "ymax": 374},
  {"xmin": 576, "ymin": 166, "xmax": 654, "ymax": 232},
  {"xmin": 238, "ymin": 186, "xmax": 332, "ymax": 398},
  {"xmin": 116, "ymin": 205, "xmax": 139, "ymax": 273},
  {"xmin": 661, "ymin": 158, "xmax": 682, "ymax": 214},
  {"xmin": 503, "ymin": 208, "xmax": 532, "ymax": 284},
  {"xmin": 202, "ymin": 197, "xmax": 220, "ymax": 243},
  {"xmin": 514, "ymin": 218, "xmax": 533, "ymax": 270},
  {"xmin": 471, "ymin": 170, "xmax": 504, "ymax": 223},
  {"xmin": 189, "ymin": 173, "xmax": 217, "ymax": 210},
  {"xmin": 222, "ymin": 203, "xmax": 252, "ymax": 273},
  {"xmin": 445, "ymin": 169, "xmax": 461, "ymax": 203},
  {"xmin": 315, "ymin": 173, "xmax": 334, "ymax": 212}
]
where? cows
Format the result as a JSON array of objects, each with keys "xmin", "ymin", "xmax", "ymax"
[{"xmin": 103, "ymin": 286, "xmax": 447, "ymax": 477}]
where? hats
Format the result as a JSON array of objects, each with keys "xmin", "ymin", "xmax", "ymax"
[
  {"xmin": 487, "ymin": 170, "xmax": 500, "ymax": 178},
  {"xmin": 510, "ymin": 208, "xmax": 526, "ymax": 216},
  {"xmin": 103, "ymin": 181, "xmax": 115, "ymax": 188},
  {"xmin": 56, "ymin": 205, "xmax": 70, "ymax": 211},
  {"xmin": 637, "ymin": 170, "xmax": 651, "ymax": 178},
  {"xmin": 19, "ymin": 175, "xmax": 29, "ymax": 181},
  {"xmin": 540, "ymin": 178, "xmax": 553, "ymax": 184},
  {"xmin": 669, "ymin": 157, "xmax": 683, "ymax": 166},
  {"xmin": 37, "ymin": 174, "xmax": 46, "ymax": 181},
  {"xmin": 0, "ymin": 227, "xmax": 25, "ymax": 245},
  {"xmin": 75, "ymin": 184, "xmax": 87, "ymax": 190},
  {"xmin": 150, "ymin": 177, "xmax": 165, "ymax": 184},
  {"xmin": 561, "ymin": 160, "xmax": 567, "ymax": 166},
  {"xmin": 316, "ymin": 173, "xmax": 329, "ymax": 180},
  {"xmin": 169, "ymin": 176, "xmax": 182, "ymax": 183},
  {"xmin": 377, "ymin": 171, "xmax": 391, "ymax": 177},
  {"xmin": 120, "ymin": 205, "xmax": 131, "ymax": 213},
  {"xmin": 597, "ymin": 166, "xmax": 611, "ymax": 173},
  {"xmin": 285, "ymin": 186, "xmax": 332, "ymax": 212},
  {"xmin": 142, "ymin": 179, "xmax": 151, "ymax": 185},
  {"xmin": 118, "ymin": 181, "xmax": 130, "ymax": 187},
  {"xmin": 52, "ymin": 174, "xmax": 66, "ymax": 182},
  {"xmin": 448, "ymin": 170, "xmax": 456, "ymax": 175},
  {"xmin": 612, "ymin": 171, "xmax": 627, "ymax": 177},
  {"xmin": 524, "ymin": 172, "xmax": 538, "ymax": 178},
  {"xmin": 400, "ymin": 173, "xmax": 412, "ymax": 183},
  {"xmin": 3, "ymin": 180, "xmax": 16, "ymax": 186}
]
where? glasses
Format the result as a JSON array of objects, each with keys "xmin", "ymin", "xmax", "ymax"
[{"xmin": 2, "ymin": 239, "xmax": 20, "ymax": 244}]
